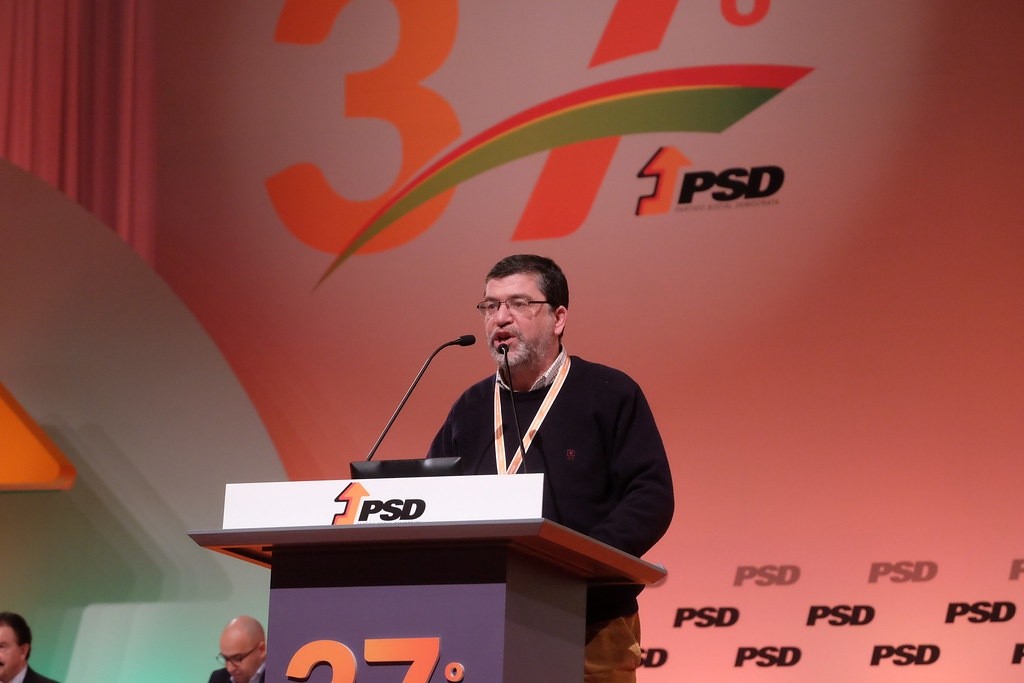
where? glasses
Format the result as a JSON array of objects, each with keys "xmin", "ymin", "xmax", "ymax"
[
  {"xmin": 216, "ymin": 643, "xmax": 259, "ymax": 666},
  {"xmin": 476, "ymin": 297, "xmax": 549, "ymax": 318}
]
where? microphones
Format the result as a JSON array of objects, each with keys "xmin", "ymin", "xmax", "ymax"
[
  {"xmin": 496, "ymin": 343, "xmax": 528, "ymax": 474},
  {"xmin": 366, "ymin": 335, "xmax": 476, "ymax": 462}
]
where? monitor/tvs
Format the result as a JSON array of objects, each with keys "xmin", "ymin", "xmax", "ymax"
[{"xmin": 349, "ymin": 457, "xmax": 464, "ymax": 480}]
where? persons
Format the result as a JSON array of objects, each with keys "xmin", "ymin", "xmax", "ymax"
[
  {"xmin": 0, "ymin": 612, "xmax": 62, "ymax": 683},
  {"xmin": 208, "ymin": 616, "xmax": 266, "ymax": 683},
  {"xmin": 426, "ymin": 254, "xmax": 675, "ymax": 682}
]
